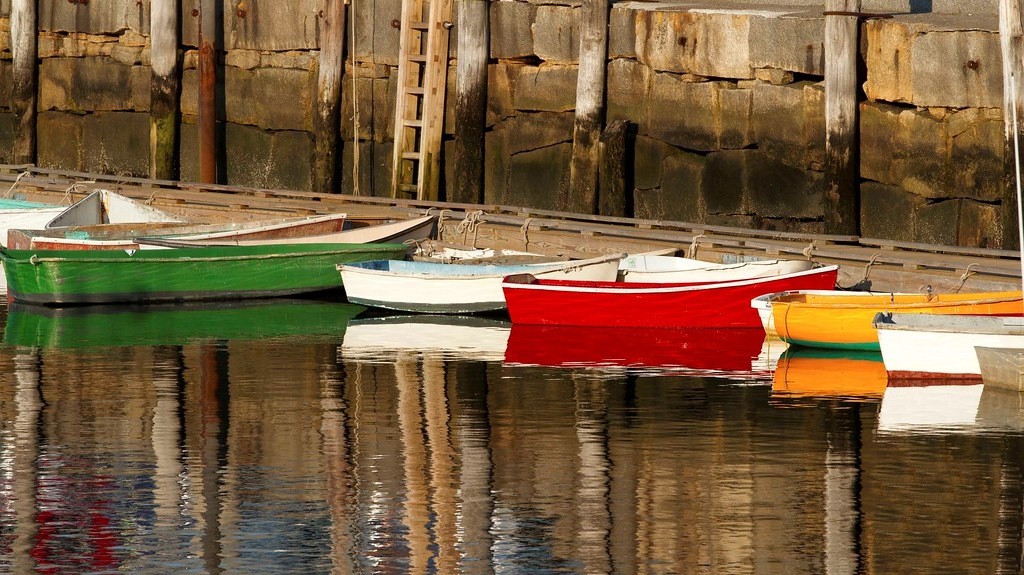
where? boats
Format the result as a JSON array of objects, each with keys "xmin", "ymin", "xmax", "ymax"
[
  {"xmin": 0, "ymin": 294, "xmax": 390, "ymax": 356},
  {"xmin": 498, "ymin": 322, "xmax": 779, "ymax": 390},
  {"xmin": 1, "ymin": 189, "xmax": 442, "ymax": 308},
  {"xmin": 973, "ymin": 344, "xmax": 1024, "ymax": 393},
  {"xmin": 337, "ymin": 246, "xmax": 688, "ymax": 318},
  {"xmin": 336, "ymin": 305, "xmax": 511, "ymax": 367},
  {"xmin": 751, "ymin": 288, "xmax": 1023, "ymax": 354},
  {"xmin": 972, "ymin": 383, "xmax": 1024, "ymax": 438},
  {"xmin": 875, "ymin": 378, "xmax": 986, "ymax": 437},
  {"xmin": 503, "ymin": 258, "xmax": 843, "ymax": 333},
  {"xmin": 769, "ymin": 343, "xmax": 888, "ymax": 410},
  {"xmin": 872, "ymin": 310, "xmax": 1024, "ymax": 383}
]
